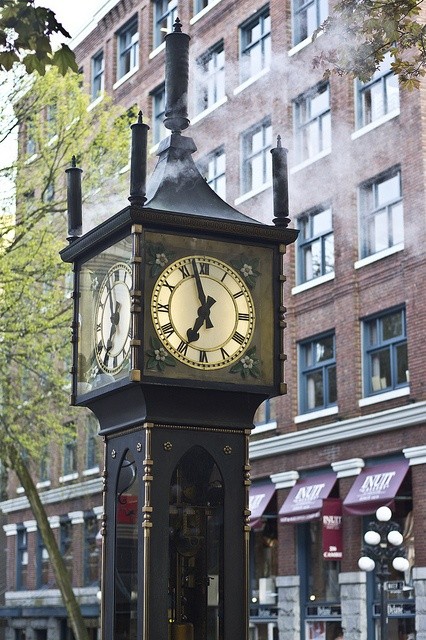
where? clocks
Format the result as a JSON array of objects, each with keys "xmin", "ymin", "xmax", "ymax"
[
  {"xmin": 72, "ymin": 235, "xmax": 136, "ymax": 396},
  {"xmin": 137, "ymin": 220, "xmax": 284, "ymax": 396}
]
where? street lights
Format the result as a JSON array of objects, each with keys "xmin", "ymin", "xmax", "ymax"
[{"xmin": 357, "ymin": 506, "xmax": 410, "ymax": 640}]
